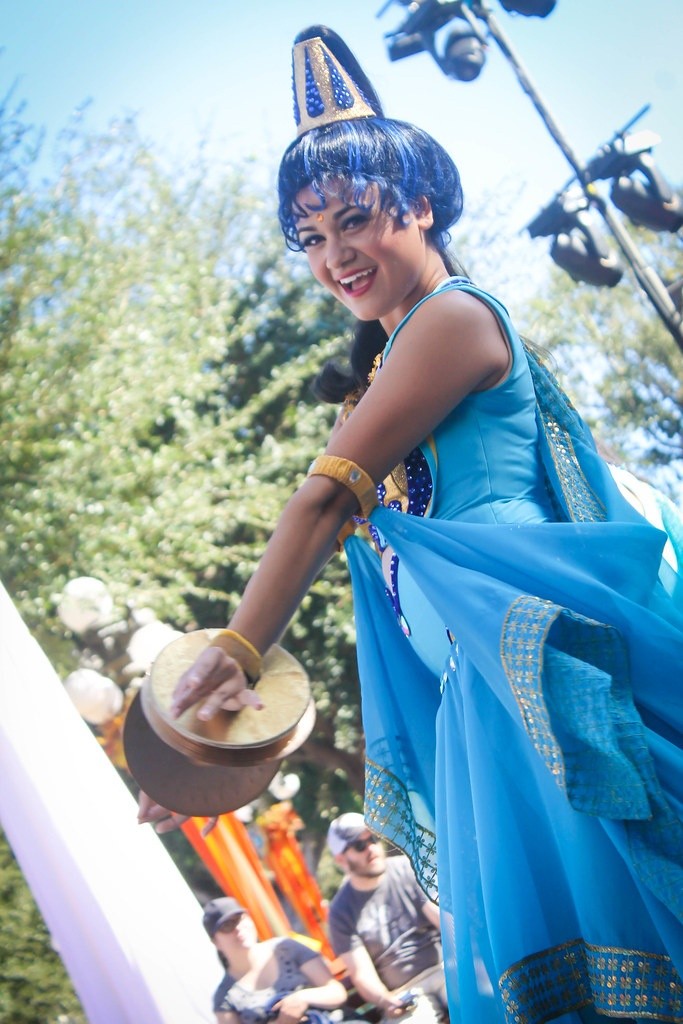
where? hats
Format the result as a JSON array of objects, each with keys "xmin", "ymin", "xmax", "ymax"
[
  {"xmin": 328, "ymin": 812, "xmax": 368, "ymax": 856},
  {"xmin": 203, "ymin": 897, "xmax": 245, "ymax": 937}
]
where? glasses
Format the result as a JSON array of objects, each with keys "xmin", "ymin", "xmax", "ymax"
[
  {"xmin": 343, "ymin": 833, "xmax": 380, "ymax": 854},
  {"xmin": 218, "ymin": 918, "xmax": 239, "ymax": 933}
]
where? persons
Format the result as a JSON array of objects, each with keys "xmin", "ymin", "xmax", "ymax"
[
  {"xmin": 204, "ymin": 896, "xmax": 348, "ymax": 1024},
  {"xmin": 326, "ymin": 811, "xmax": 494, "ymax": 1024},
  {"xmin": 137, "ymin": 24, "xmax": 683, "ymax": 1024}
]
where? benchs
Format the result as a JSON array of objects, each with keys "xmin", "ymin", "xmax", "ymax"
[{"xmin": 338, "ymin": 976, "xmax": 449, "ymax": 1023}]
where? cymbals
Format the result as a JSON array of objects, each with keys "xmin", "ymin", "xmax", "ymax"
[{"xmin": 124, "ymin": 630, "xmax": 312, "ymax": 814}]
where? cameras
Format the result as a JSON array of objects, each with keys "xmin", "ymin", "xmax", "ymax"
[{"xmin": 394, "ymin": 990, "xmax": 421, "ymax": 1011}]
